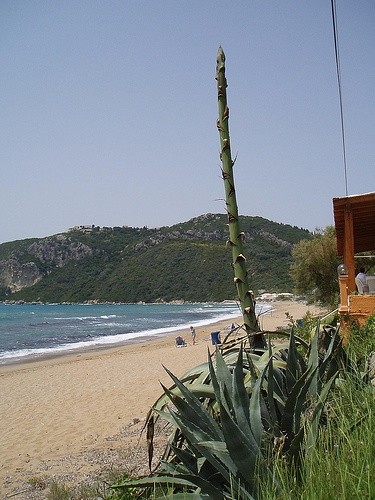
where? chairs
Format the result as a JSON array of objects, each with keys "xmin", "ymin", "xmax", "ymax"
[{"xmin": 355, "ymin": 278, "xmax": 368, "ymax": 294}]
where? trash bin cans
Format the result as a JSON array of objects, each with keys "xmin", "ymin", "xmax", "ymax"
[{"xmin": 211, "ymin": 331, "xmax": 222, "ymax": 345}]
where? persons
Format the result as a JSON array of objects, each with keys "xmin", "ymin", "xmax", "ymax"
[
  {"xmin": 356, "ymin": 267, "xmax": 367, "ymax": 285},
  {"xmin": 190, "ymin": 326, "xmax": 196, "ymax": 344}
]
